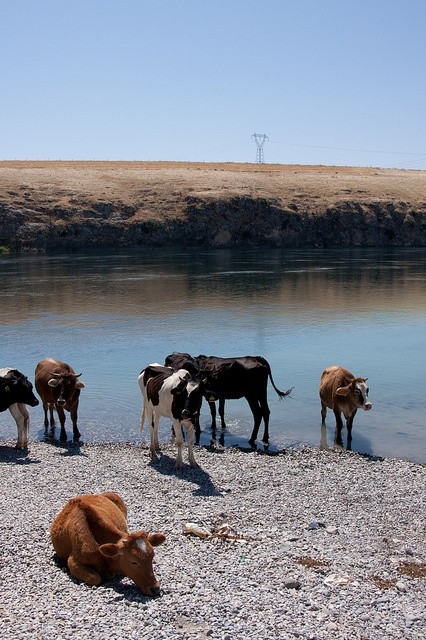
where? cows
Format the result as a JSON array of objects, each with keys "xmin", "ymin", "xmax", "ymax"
[
  {"xmin": 163, "ymin": 351, "xmax": 217, "ymax": 446},
  {"xmin": 192, "ymin": 354, "xmax": 295, "ymax": 442},
  {"xmin": 33, "ymin": 356, "xmax": 84, "ymax": 435},
  {"xmin": 0, "ymin": 367, "xmax": 30, "ymax": 449},
  {"xmin": 48, "ymin": 490, "xmax": 166, "ymax": 596},
  {"xmin": 135, "ymin": 362, "xmax": 207, "ymax": 469},
  {"xmin": 317, "ymin": 363, "xmax": 372, "ymax": 451},
  {"xmin": 0, "ymin": 369, "xmax": 39, "ymax": 413}
]
